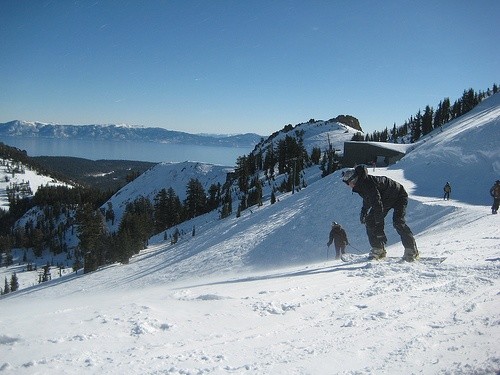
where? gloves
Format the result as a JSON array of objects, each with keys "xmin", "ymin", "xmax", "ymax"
[
  {"xmin": 372, "ymin": 223, "xmax": 387, "ymax": 245},
  {"xmin": 360, "ymin": 207, "xmax": 368, "ymax": 224}
]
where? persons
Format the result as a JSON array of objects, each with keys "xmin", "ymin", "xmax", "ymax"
[
  {"xmin": 341, "ymin": 164, "xmax": 418, "ymax": 261},
  {"xmin": 442, "ymin": 182, "xmax": 452, "ymax": 200},
  {"xmin": 489, "ymin": 179, "xmax": 500, "ymax": 214},
  {"xmin": 327, "ymin": 222, "xmax": 348, "ymax": 257}
]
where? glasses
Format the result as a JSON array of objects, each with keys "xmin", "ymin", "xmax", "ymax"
[{"xmin": 342, "ymin": 173, "xmax": 357, "ymax": 185}]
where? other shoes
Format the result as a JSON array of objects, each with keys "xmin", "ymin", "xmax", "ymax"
[
  {"xmin": 368, "ymin": 246, "xmax": 386, "ymax": 260},
  {"xmin": 492, "ymin": 210, "xmax": 497, "ymax": 214},
  {"xmin": 396, "ymin": 248, "xmax": 419, "ymax": 265}
]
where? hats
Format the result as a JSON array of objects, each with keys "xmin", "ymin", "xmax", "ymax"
[
  {"xmin": 331, "ymin": 221, "xmax": 338, "ymax": 226},
  {"xmin": 341, "ymin": 170, "xmax": 354, "ymax": 180}
]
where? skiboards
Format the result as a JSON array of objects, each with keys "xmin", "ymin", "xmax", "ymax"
[{"xmin": 340, "ymin": 253, "xmax": 446, "ymax": 267}]
